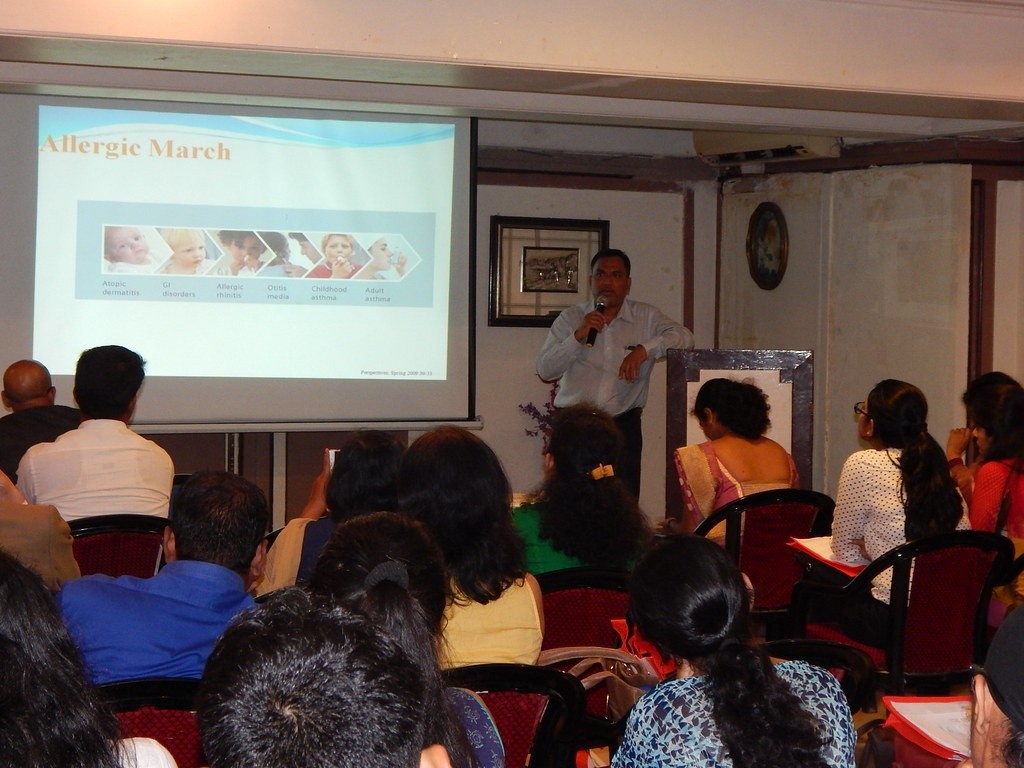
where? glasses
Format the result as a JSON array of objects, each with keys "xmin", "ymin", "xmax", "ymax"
[{"xmin": 854, "ymin": 402, "xmax": 875, "ymax": 422}]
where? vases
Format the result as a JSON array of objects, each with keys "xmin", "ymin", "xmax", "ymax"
[{"xmin": 542, "ymin": 427, "xmax": 555, "ymax": 481}]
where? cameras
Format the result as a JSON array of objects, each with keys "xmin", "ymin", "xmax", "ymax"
[{"xmin": 329, "ymin": 450, "xmax": 340, "ymax": 470}]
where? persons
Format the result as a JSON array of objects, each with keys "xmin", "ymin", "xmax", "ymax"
[
  {"xmin": 55, "ymin": 472, "xmax": 268, "ymax": 678},
  {"xmin": 262, "ymin": 429, "xmax": 410, "ymax": 584},
  {"xmin": 198, "ymin": 511, "xmax": 504, "ymax": 768},
  {"xmin": 16, "ymin": 346, "xmax": 175, "ymax": 518},
  {"xmin": 0, "ymin": 471, "xmax": 79, "ymax": 591},
  {"xmin": 655, "ymin": 378, "xmax": 799, "ymax": 548},
  {"xmin": 949, "ymin": 372, "xmax": 1024, "ymax": 604},
  {"xmin": 0, "ymin": 548, "xmax": 177, "ymax": 768},
  {"xmin": 537, "ymin": 249, "xmax": 694, "ymax": 505},
  {"xmin": 396, "ymin": 427, "xmax": 544, "ymax": 672},
  {"xmin": 967, "ymin": 604, "xmax": 1024, "ymax": 768},
  {"xmin": 0, "ymin": 360, "xmax": 85, "ymax": 485},
  {"xmin": 105, "ymin": 226, "xmax": 407, "ymax": 281},
  {"xmin": 512, "ymin": 406, "xmax": 651, "ymax": 573},
  {"xmin": 605, "ymin": 533, "xmax": 857, "ymax": 767},
  {"xmin": 790, "ymin": 379, "xmax": 969, "ymax": 643}
]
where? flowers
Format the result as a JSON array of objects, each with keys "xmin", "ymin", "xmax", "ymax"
[{"xmin": 518, "ymin": 374, "xmax": 561, "ymax": 456}]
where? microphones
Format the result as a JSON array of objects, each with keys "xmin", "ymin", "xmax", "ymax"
[{"xmin": 586, "ymin": 296, "xmax": 609, "ymax": 347}]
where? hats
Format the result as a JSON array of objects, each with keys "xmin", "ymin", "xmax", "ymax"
[{"xmin": 985, "ymin": 604, "xmax": 1024, "ymax": 731}]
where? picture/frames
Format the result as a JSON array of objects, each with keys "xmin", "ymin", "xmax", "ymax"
[
  {"xmin": 488, "ymin": 215, "xmax": 610, "ymax": 328},
  {"xmin": 745, "ymin": 202, "xmax": 790, "ymax": 290}
]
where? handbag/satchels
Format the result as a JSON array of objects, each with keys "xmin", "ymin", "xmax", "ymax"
[{"xmin": 536, "ymin": 647, "xmax": 660, "ymax": 724}]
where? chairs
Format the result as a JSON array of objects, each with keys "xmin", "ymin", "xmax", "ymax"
[{"xmin": 66, "ymin": 489, "xmax": 1024, "ymax": 768}]
